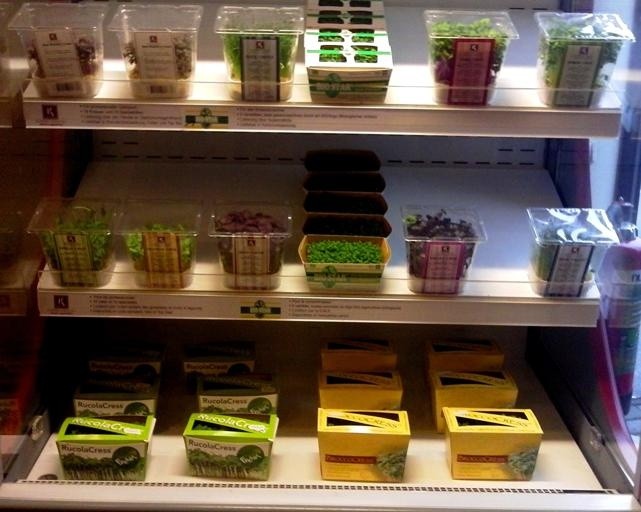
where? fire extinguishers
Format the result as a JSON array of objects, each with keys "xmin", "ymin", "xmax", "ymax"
[{"xmin": 595, "ymin": 198, "xmax": 641, "ymax": 417}]
